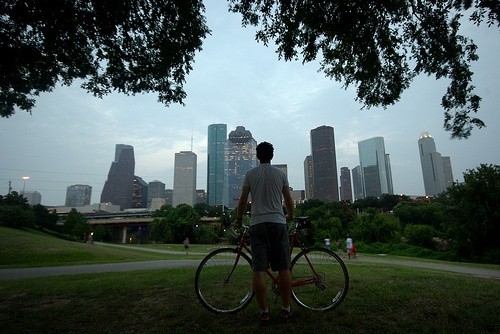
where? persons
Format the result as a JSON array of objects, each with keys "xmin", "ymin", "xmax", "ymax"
[
  {"xmin": 235, "ymin": 142, "xmax": 299, "ymax": 320},
  {"xmin": 325, "ymin": 237, "xmax": 331, "ymax": 250},
  {"xmin": 346, "ymin": 233, "xmax": 356, "ymax": 259},
  {"xmin": 183, "ymin": 237, "xmax": 189, "ymax": 255},
  {"xmin": 89, "ymin": 232, "xmax": 94, "ymax": 244}
]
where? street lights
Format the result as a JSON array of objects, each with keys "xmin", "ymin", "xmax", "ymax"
[{"xmin": 22, "ymin": 177, "xmax": 30, "ymax": 195}]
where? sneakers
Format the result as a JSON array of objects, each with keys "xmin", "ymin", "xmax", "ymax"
[
  {"xmin": 255, "ymin": 310, "xmax": 270, "ymax": 322},
  {"xmin": 278, "ymin": 308, "xmax": 292, "ymax": 320}
]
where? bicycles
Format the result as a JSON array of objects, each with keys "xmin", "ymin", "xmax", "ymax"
[{"xmin": 194, "ymin": 215, "xmax": 349, "ymax": 314}]
